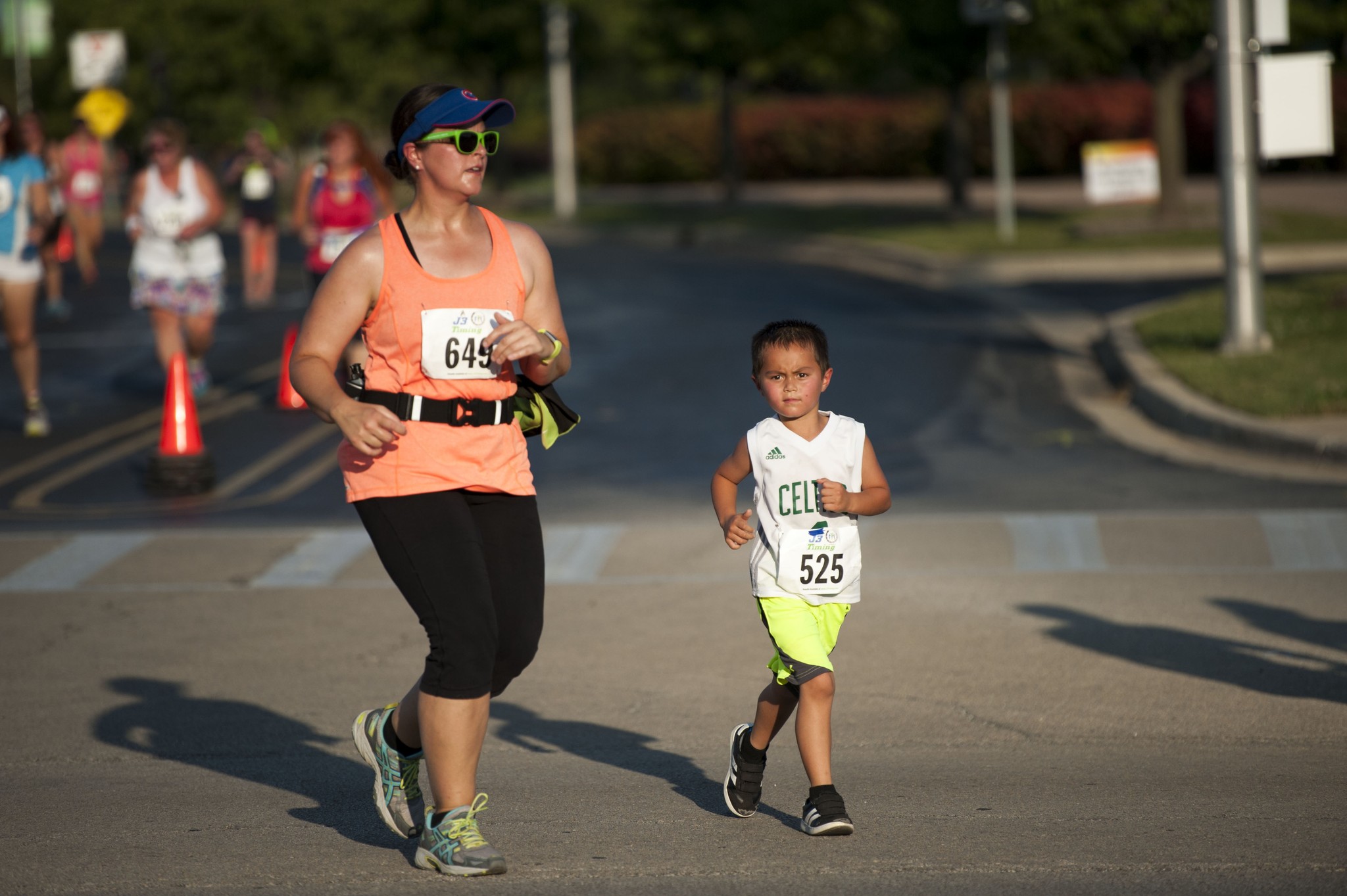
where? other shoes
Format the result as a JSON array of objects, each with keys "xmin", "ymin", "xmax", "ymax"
[
  {"xmin": 188, "ymin": 360, "xmax": 207, "ymax": 401},
  {"xmin": 49, "ymin": 299, "xmax": 73, "ymax": 322},
  {"xmin": 26, "ymin": 404, "xmax": 50, "ymax": 438}
]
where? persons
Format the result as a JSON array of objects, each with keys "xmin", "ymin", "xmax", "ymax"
[
  {"xmin": 287, "ymin": 82, "xmax": 574, "ymax": 877},
  {"xmin": 290, "ymin": 119, "xmax": 395, "ymax": 402},
  {"xmin": 1, "ymin": 105, "xmax": 114, "ymax": 439},
  {"xmin": 124, "ymin": 120, "xmax": 228, "ymax": 402},
  {"xmin": 220, "ymin": 131, "xmax": 295, "ymax": 307},
  {"xmin": 710, "ymin": 318, "xmax": 892, "ymax": 838}
]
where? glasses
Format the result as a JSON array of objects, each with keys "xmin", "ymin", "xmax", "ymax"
[{"xmin": 416, "ymin": 131, "xmax": 500, "ymax": 155}]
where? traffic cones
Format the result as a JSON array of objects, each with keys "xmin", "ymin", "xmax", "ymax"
[
  {"xmin": 269, "ymin": 324, "xmax": 311, "ymax": 412},
  {"xmin": 141, "ymin": 351, "xmax": 220, "ymax": 487}
]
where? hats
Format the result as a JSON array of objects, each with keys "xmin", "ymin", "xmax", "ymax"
[{"xmin": 397, "ymin": 87, "xmax": 516, "ymax": 163}]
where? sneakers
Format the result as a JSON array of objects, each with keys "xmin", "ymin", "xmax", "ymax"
[
  {"xmin": 801, "ymin": 792, "xmax": 855, "ymax": 836},
  {"xmin": 413, "ymin": 792, "xmax": 507, "ymax": 877},
  {"xmin": 350, "ymin": 703, "xmax": 425, "ymax": 840},
  {"xmin": 723, "ymin": 724, "xmax": 767, "ymax": 818}
]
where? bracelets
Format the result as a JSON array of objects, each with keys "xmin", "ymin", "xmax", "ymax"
[{"xmin": 538, "ymin": 328, "xmax": 562, "ymax": 365}]
